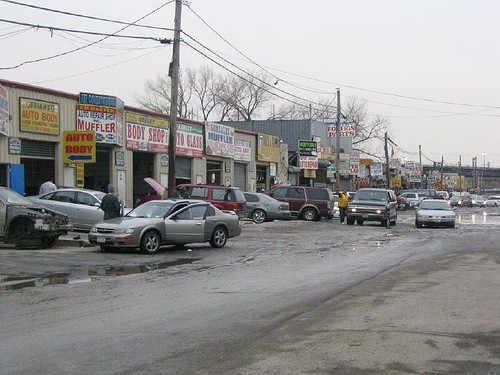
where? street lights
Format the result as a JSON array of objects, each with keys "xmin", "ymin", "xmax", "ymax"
[
  {"xmin": 472, "ymin": 156, "xmax": 477, "ymax": 190},
  {"xmin": 483, "ymin": 151, "xmax": 487, "ymax": 171}
]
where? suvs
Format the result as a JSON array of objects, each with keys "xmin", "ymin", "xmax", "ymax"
[
  {"xmin": 264, "ymin": 185, "xmax": 335, "ymax": 222},
  {"xmin": 332, "ymin": 188, "xmax": 399, "ymax": 228}
]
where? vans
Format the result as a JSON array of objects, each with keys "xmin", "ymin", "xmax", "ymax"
[{"xmin": 135, "ymin": 178, "xmax": 248, "ymax": 216}]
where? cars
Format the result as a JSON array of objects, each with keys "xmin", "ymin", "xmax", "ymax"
[
  {"xmin": 413, "ymin": 200, "xmax": 457, "ymax": 228},
  {"xmin": 25, "ymin": 187, "xmax": 133, "ymax": 231},
  {"xmin": 396, "ymin": 188, "xmax": 449, "ymax": 211},
  {"xmin": 0, "ymin": 186, "xmax": 72, "ymax": 247},
  {"xmin": 242, "ymin": 192, "xmax": 292, "ymax": 224},
  {"xmin": 448, "ymin": 188, "xmax": 500, "ymax": 208},
  {"xmin": 88, "ymin": 200, "xmax": 243, "ymax": 255}
]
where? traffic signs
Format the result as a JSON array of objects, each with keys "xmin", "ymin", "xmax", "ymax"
[{"xmin": 62, "ymin": 130, "xmax": 97, "ymax": 164}]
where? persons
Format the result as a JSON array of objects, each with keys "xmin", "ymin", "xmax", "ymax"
[
  {"xmin": 179, "ymin": 186, "xmax": 191, "ymax": 199},
  {"xmin": 39, "ymin": 177, "xmax": 57, "ymax": 195},
  {"xmin": 397, "ymin": 194, "xmax": 406, "ymax": 210},
  {"xmin": 101, "ymin": 184, "xmax": 120, "ymax": 220},
  {"xmin": 338, "ymin": 190, "xmax": 350, "ymax": 224}
]
